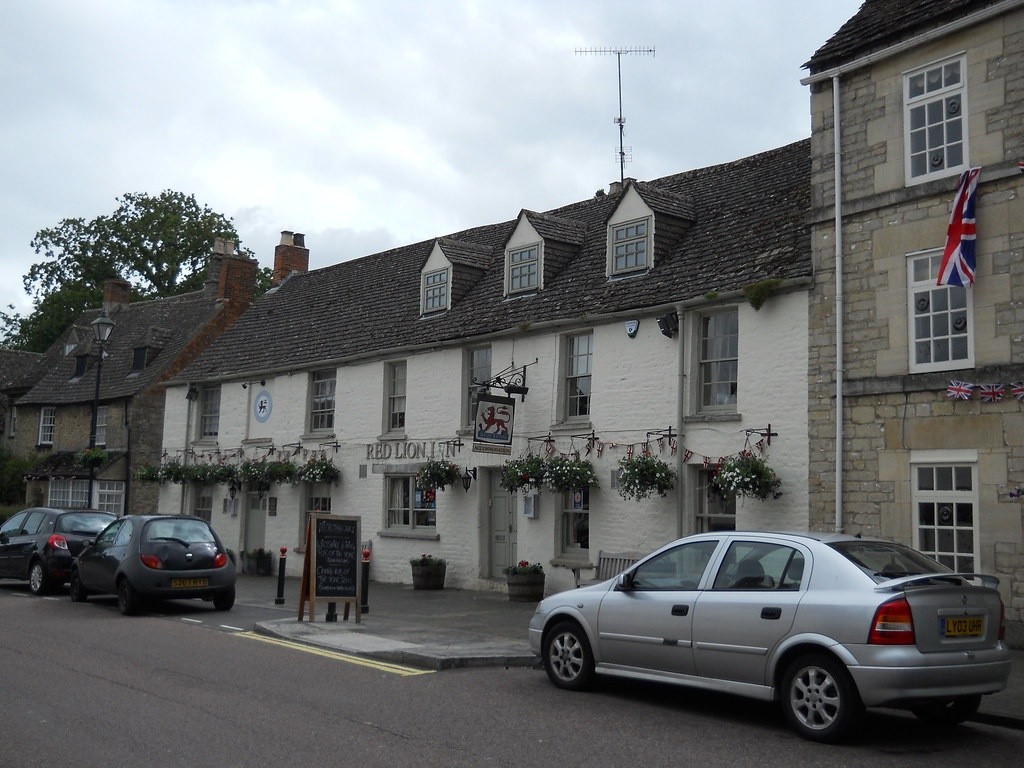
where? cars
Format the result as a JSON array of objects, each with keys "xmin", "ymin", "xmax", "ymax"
[
  {"xmin": 0, "ymin": 504, "xmax": 123, "ymax": 598},
  {"xmin": 527, "ymin": 529, "xmax": 1012, "ymax": 744},
  {"xmin": 67, "ymin": 512, "xmax": 238, "ymax": 617}
]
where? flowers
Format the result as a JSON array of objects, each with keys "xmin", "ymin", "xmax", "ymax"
[
  {"xmin": 503, "ymin": 559, "xmax": 543, "ymax": 574},
  {"xmin": 248, "ymin": 547, "xmax": 268, "ymax": 557},
  {"xmin": 412, "ymin": 458, "xmax": 459, "ymax": 491},
  {"xmin": 297, "ymin": 458, "xmax": 337, "ymax": 484},
  {"xmin": 498, "ymin": 456, "xmax": 545, "ymax": 491},
  {"xmin": 619, "ymin": 456, "xmax": 676, "ymax": 498},
  {"xmin": 82, "ymin": 444, "xmax": 107, "ymax": 460},
  {"xmin": 410, "ymin": 553, "xmax": 443, "ymax": 566},
  {"xmin": 713, "ymin": 455, "xmax": 780, "ymax": 503},
  {"xmin": 130, "ymin": 458, "xmax": 295, "ymax": 481},
  {"xmin": 541, "ymin": 455, "xmax": 601, "ymax": 493}
]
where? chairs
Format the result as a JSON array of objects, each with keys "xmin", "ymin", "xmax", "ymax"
[
  {"xmin": 787, "ymin": 557, "xmax": 804, "ymax": 589},
  {"xmin": 736, "ymin": 558, "xmax": 765, "ymax": 588}
]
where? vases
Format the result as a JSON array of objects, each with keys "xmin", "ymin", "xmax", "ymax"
[
  {"xmin": 83, "ymin": 459, "xmax": 101, "ymax": 467},
  {"xmin": 413, "ymin": 566, "xmax": 446, "ymax": 591},
  {"xmin": 507, "ymin": 577, "xmax": 545, "ymax": 602},
  {"xmin": 243, "ymin": 557, "xmax": 271, "ymax": 577}
]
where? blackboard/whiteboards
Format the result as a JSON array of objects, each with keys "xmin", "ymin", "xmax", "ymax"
[{"xmin": 300, "ymin": 512, "xmax": 362, "ymax": 602}]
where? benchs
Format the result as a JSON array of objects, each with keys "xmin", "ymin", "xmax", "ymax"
[{"xmin": 571, "ymin": 551, "xmax": 645, "ymax": 591}]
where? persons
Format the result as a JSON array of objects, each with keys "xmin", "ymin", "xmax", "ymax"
[{"xmin": 733, "ymin": 560, "xmax": 765, "ymax": 589}]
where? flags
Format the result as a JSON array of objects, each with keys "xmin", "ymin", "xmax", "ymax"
[
  {"xmin": 936, "ymin": 166, "xmax": 982, "ymax": 285},
  {"xmin": 946, "ymin": 380, "xmax": 975, "ymax": 400},
  {"xmin": 980, "ymin": 384, "xmax": 1007, "ymax": 403},
  {"xmin": 1011, "ymin": 382, "xmax": 1024, "ymax": 400}
]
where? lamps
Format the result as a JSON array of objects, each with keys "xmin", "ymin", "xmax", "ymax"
[
  {"xmin": 462, "ymin": 466, "xmax": 477, "ymax": 491},
  {"xmin": 657, "ymin": 312, "xmax": 680, "ymax": 340},
  {"xmin": 257, "ymin": 480, "xmax": 271, "ymax": 501},
  {"xmin": 228, "ymin": 481, "xmax": 241, "ymax": 500},
  {"xmin": 242, "ymin": 379, "xmax": 265, "ymax": 390},
  {"xmin": 185, "ymin": 389, "xmax": 199, "ymax": 399}
]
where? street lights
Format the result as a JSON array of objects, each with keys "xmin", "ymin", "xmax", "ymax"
[{"xmin": 87, "ymin": 307, "xmax": 116, "ymax": 508}]
